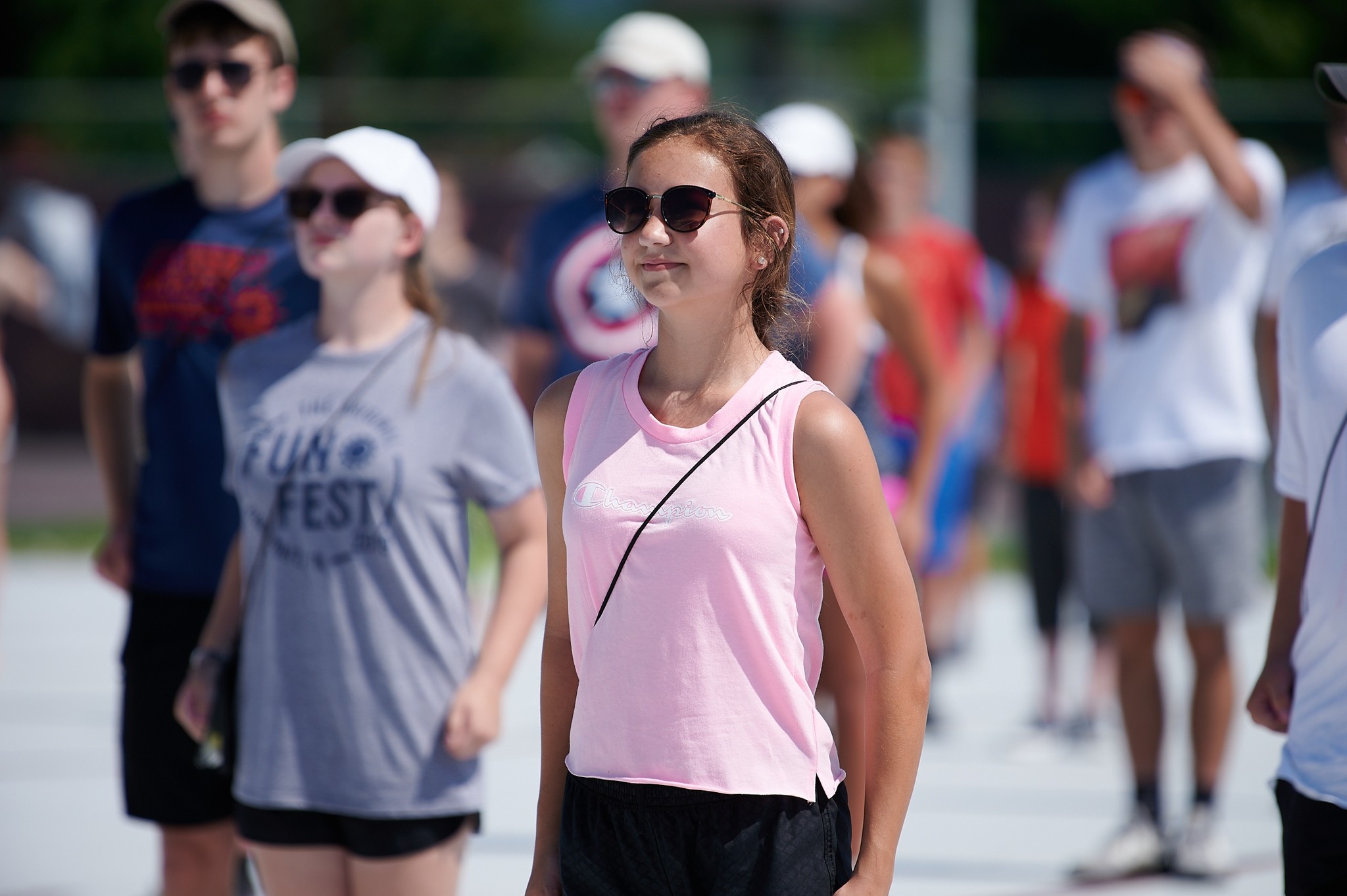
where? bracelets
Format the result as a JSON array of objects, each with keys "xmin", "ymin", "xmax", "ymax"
[{"xmin": 191, "ymin": 649, "xmax": 226, "ymax": 671}]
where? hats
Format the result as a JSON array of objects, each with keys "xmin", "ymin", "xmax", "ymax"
[
  {"xmin": 273, "ymin": 125, "xmax": 440, "ymax": 234},
  {"xmin": 578, "ymin": 11, "xmax": 710, "ymax": 85},
  {"xmin": 155, "ymin": 0, "xmax": 299, "ymax": 66},
  {"xmin": 756, "ymin": 103, "xmax": 856, "ymax": 183}
]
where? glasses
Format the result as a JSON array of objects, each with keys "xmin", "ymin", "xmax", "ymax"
[
  {"xmin": 171, "ymin": 60, "xmax": 255, "ymax": 92},
  {"xmin": 600, "ymin": 181, "xmax": 764, "ymax": 236},
  {"xmin": 284, "ymin": 183, "xmax": 391, "ymax": 222}
]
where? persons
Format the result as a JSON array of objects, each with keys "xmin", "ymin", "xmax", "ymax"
[
  {"xmin": 751, "ymin": 100, "xmax": 948, "ymax": 607},
  {"xmin": 502, "ymin": 11, "xmax": 862, "ymax": 426},
  {"xmin": 525, "ymin": 112, "xmax": 933, "ymax": 896},
  {"xmin": 860, "ymin": 125, "xmax": 1116, "ymax": 739},
  {"xmin": 1246, "ymin": 61, "xmax": 1347, "ymax": 896},
  {"xmin": 86, "ymin": 0, "xmax": 321, "ymax": 896},
  {"xmin": 1040, "ymin": 33, "xmax": 1287, "ymax": 881},
  {"xmin": 175, "ymin": 126, "xmax": 547, "ymax": 896}
]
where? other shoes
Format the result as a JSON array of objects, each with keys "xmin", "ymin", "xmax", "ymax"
[
  {"xmin": 1170, "ymin": 801, "xmax": 1238, "ymax": 877},
  {"xmin": 1070, "ymin": 800, "xmax": 1175, "ymax": 883}
]
